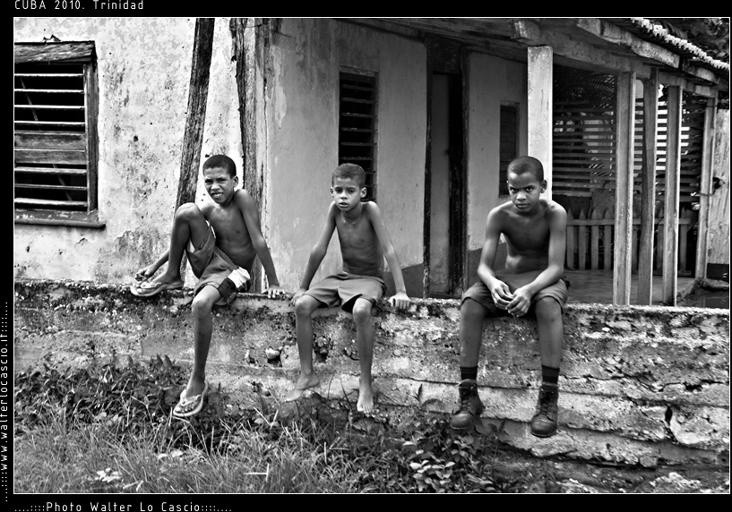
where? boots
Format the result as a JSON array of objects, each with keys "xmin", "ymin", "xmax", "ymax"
[
  {"xmin": 531, "ymin": 382, "xmax": 557, "ymax": 438},
  {"xmin": 449, "ymin": 381, "xmax": 483, "ymax": 429}
]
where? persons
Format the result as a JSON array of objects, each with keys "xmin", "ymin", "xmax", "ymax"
[
  {"xmin": 448, "ymin": 155, "xmax": 571, "ymax": 438},
  {"xmin": 130, "ymin": 155, "xmax": 285, "ymax": 418},
  {"xmin": 287, "ymin": 162, "xmax": 411, "ymax": 415}
]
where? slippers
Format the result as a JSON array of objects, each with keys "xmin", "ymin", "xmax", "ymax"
[
  {"xmin": 173, "ymin": 382, "xmax": 209, "ymax": 417},
  {"xmin": 130, "ymin": 276, "xmax": 184, "ymax": 295}
]
